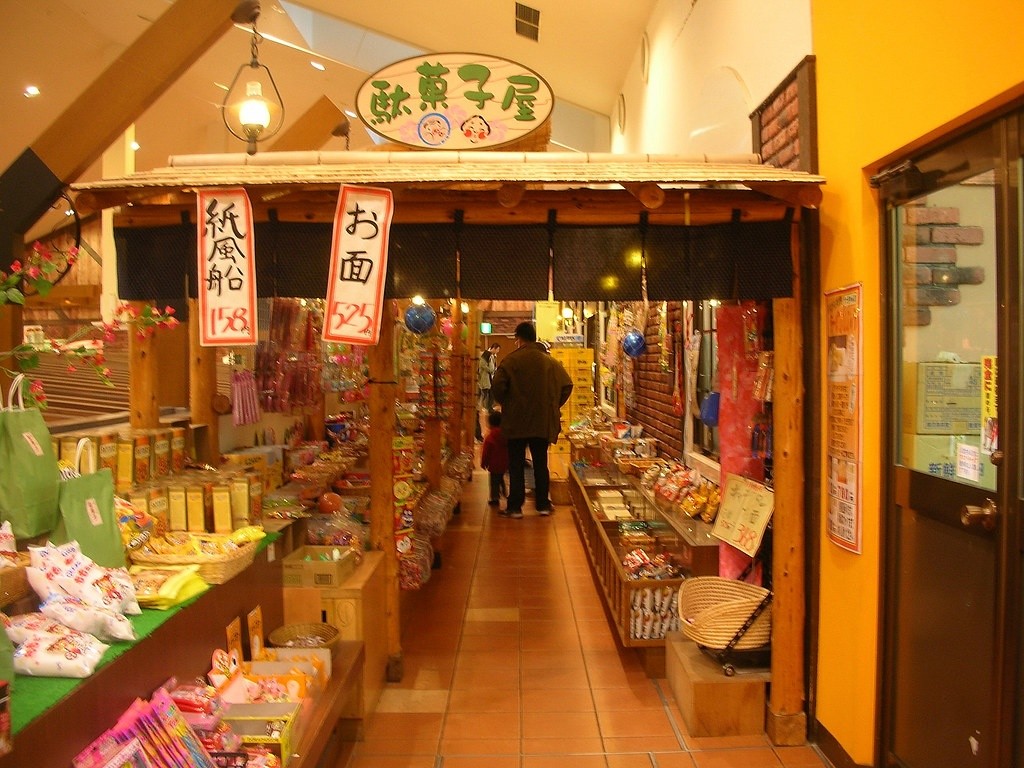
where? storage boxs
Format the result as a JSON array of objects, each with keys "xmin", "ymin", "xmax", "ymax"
[{"xmin": 50, "ymin": 426, "xmax": 354, "ymax": 768}]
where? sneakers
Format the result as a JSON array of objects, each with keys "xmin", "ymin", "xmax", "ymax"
[
  {"xmin": 536, "ymin": 503, "xmax": 554, "ymax": 516},
  {"xmin": 497, "ymin": 508, "xmax": 524, "ymax": 519}
]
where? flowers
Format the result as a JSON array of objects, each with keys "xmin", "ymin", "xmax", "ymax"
[{"xmin": 0, "ymin": 241, "xmax": 179, "ymax": 410}]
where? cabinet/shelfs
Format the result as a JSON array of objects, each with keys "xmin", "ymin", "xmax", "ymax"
[{"xmin": 282, "ymin": 552, "xmax": 387, "ymax": 740}]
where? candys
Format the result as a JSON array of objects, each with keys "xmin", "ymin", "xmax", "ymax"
[
  {"xmin": 264, "ymin": 449, "xmax": 430, "ymax": 587},
  {"xmin": 322, "ymin": 344, "xmax": 367, "ymax": 389}
]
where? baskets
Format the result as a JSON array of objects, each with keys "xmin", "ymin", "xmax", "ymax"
[
  {"xmin": 268, "ymin": 621, "xmax": 341, "ymax": 652},
  {"xmin": 128, "ymin": 529, "xmax": 261, "ymax": 585},
  {"xmin": 677, "ymin": 576, "xmax": 774, "ymax": 650},
  {"xmin": 549, "ymin": 471, "xmax": 572, "ymax": 505}
]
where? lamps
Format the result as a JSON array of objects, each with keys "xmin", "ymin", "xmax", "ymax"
[{"xmin": 221, "ymin": 0, "xmax": 285, "ymax": 154}]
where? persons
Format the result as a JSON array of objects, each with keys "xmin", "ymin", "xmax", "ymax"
[
  {"xmin": 490, "ymin": 321, "xmax": 573, "ymax": 518},
  {"xmin": 477, "ymin": 342, "xmax": 501, "ymax": 411},
  {"xmin": 479, "ymin": 411, "xmax": 508, "ymax": 506}
]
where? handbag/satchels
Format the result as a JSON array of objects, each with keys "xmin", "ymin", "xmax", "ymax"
[
  {"xmin": 0, "ymin": 372, "xmax": 60, "ymax": 539},
  {"xmin": 50, "ymin": 437, "xmax": 128, "ymax": 571}
]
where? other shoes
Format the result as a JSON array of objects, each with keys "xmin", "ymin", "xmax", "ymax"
[{"xmin": 487, "ymin": 498, "xmax": 499, "ymax": 505}]
